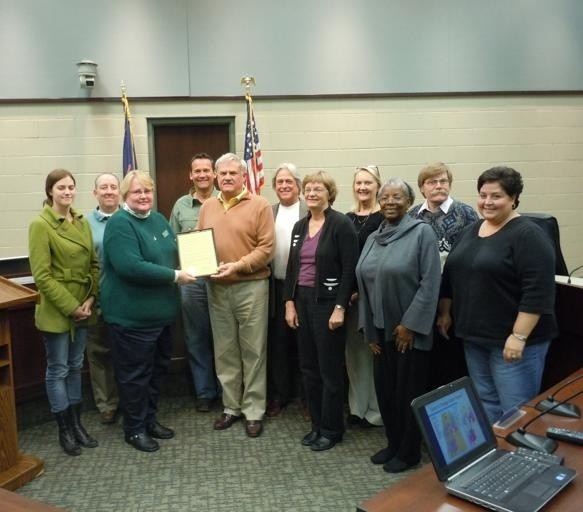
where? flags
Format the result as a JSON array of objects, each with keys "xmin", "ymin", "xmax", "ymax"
[
  {"xmin": 122, "ymin": 109, "xmax": 138, "ymax": 179},
  {"xmin": 243, "ymin": 96, "xmax": 264, "ymax": 196}
]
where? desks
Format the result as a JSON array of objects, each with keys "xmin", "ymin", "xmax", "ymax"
[{"xmin": 356, "ymin": 367, "xmax": 582, "ymax": 511}]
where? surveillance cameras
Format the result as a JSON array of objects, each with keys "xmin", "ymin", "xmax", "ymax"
[{"xmin": 80, "ymin": 75, "xmax": 95, "ymax": 88}]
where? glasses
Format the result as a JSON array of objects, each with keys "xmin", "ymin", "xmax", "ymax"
[
  {"xmin": 355, "ymin": 165, "xmax": 379, "ymax": 174},
  {"xmin": 379, "ymin": 195, "xmax": 410, "ymax": 204}
]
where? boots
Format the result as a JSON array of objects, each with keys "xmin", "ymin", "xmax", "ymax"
[
  {"xmin": 53, "ymin": 404, "xmax": 83, "ymax": 455},
  {"xmin": 71, "ymin": 402, "xmax": 98, "ymax": 447}
]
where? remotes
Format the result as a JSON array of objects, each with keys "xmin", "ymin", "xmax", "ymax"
[
  {"xmin": 516, "ymin": 446, "xmax": 564, "ymax": 466},
  {"xmin": 546, "ymin": 426, "xmax": 583, "ymax": 447}
]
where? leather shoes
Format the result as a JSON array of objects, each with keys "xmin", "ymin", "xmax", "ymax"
[
  {"xmin": 371, "ymin": 448, "xmax": 392, "ymax": 463},
  {"xmin": 125, "ymin": 430, "xmax": 160, "ymax": 452},
  {"xmin": 98, "ymin": 409, "xmax": 116, "ymax": 424},
  {"xmin": 347, "ymin": 415, "xmax": 371, "ymax": 427},
  {"xmin": 146, "ymin": 420, "xmax": 174, "ymax": 439},
  {"xmin": 196, "ymin": 397, "xmax": 282, "ymax": 437},
  {"xmin": 302, "ymin": 432, "xmax": 344, "ymax": 451},
  {"xmin": 384, "ymin": 458, "xmax": 409, "ymax": 473}
]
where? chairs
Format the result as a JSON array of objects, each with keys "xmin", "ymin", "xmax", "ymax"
[{"xmin": 516, "ymin": 212, "xmax": 570, "ymax": 280}]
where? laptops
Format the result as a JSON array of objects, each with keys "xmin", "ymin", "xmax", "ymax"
[{"xmin": 411, "ymin": 376, "xmax": 576, "ymax": 512}]
